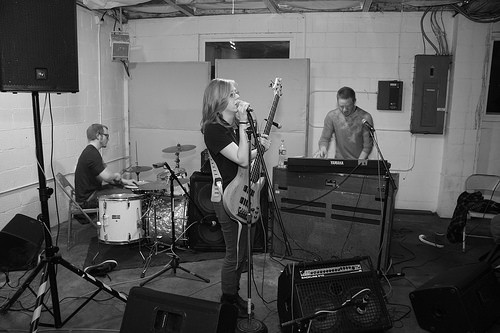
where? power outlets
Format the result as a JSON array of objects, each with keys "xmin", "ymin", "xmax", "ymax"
[{"xmin": 95, "ymin": 16, "xmax": 103, "ymax": 25}]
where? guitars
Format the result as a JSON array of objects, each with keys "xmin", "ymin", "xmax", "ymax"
[{"xmin": 222, "ymin": 78, "xmax": 285, "ymax": 225}]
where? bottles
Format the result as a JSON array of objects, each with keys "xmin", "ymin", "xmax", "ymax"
[{"xmin": 278, "ymin": 140, "xmax": 287, "ymax": 166}]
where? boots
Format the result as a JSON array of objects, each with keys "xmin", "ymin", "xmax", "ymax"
[{"xmin": 220, "ymin": 293, "xmax": 255, "ymax": 319}]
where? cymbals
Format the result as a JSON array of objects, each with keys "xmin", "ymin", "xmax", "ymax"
[
  {"xmin": 161, "ymin": 145, "xmax": 196, "ymax": 152},
  {"xmin": 123, "ymin": 165, "xmax": 152, "ymax": 173},
  {"xmin": 157, "ymin": 168, "xmax": 190, "ymax": 194}
]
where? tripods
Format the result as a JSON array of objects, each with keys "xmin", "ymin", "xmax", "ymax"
[
  {"xmin": 0, "ymin": 91, "xmax": 127, "ymax": 328},
  {"xmin": 138, "ymin": 162, "xmax": 210, "ymax": 287}
]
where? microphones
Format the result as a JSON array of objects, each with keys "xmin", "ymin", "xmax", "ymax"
[
  {"xmin": 237, "ymin": 101, "xmax": 254, "ymax": 112},
  {"xmin": 264, "ymin": 119, "xmax": 282, "ymax": 128},
  {"xmin": 357, "ymin": 290, "xmax": 371, "ymax": 314},
  {"xmin": 362, "ymin": 119, "xmax": 375, "ymax": 131},
  {"xmin": 198, "ymin": 221, "xmax": 216, "ymax": 226}
]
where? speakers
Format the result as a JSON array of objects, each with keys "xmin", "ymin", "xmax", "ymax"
[
  {"xmin": 409, "ymin": 242, "xmax": 500, "ymax": 333},
  {"xmin": 277, "ymin": 256, "xmax": 392, "ymax": 333},
  {"xmin": 0, "ymin": 0, "xmax": 79, "ymax": 94},
  {"xmin": 120, "ymin": 286, "xmax": 236, "ymax": 333},
  {"xmin": 186, "ymin": 170, "xmax": 268, "ymax": 251},
  {"xmin": 0, "ymin": 213, "xmax": 45, "ymax": 270}
]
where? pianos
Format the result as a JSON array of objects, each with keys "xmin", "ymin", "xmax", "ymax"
[{"xmin": 284, "ymin": 157, "xmax": 391, "ymax": 174}]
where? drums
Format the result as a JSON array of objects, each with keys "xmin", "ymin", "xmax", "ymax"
[
  {"xmin": 132, "ymin": 182, "xmax": 166, "ymax": 238},
  {"xmin": 96, "ymin": 192, "xmax": 146, "ymax": 246},
  {"xmin": 145, "ymin": 194, "xmax": 191, "ymax": 250}
]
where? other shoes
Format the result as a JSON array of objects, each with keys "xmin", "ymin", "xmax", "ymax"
[
  {"xmin": 419, "ymin": 231, "xmax": 446, "ymax": 248},
  {"xmin": 83, "ymin": 260, "xmax": 117, "ymax": 281}
]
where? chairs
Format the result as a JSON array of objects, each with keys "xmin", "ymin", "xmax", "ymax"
[
  {"xmin": 53, "ymin": 172, "xmax": 98, "ymax": 250},
  {"xmin": 461, "ymin": 173, "xmax": 500, "ymax": 252}
]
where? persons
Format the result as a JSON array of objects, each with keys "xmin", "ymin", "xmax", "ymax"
[
  {"xmin": 200, "ymin": 78, "xmax": 271, "ymax": 310},
  {"xmin": 73, "ymin": 123, "xmax": 138, "ymax": 202},
  {"xmin": 312, "ymin": 86, "xmax": 374, "ymax": 160}
]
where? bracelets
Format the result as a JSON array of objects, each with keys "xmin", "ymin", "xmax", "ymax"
[{"xmin": 239, "ymin": 121, "xmax": 249, "ymax": 124}]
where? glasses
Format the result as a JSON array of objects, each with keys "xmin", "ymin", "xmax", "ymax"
[{"xmin": 99, "ymin": 132, "xmax": 109, "ymax": 139}]
